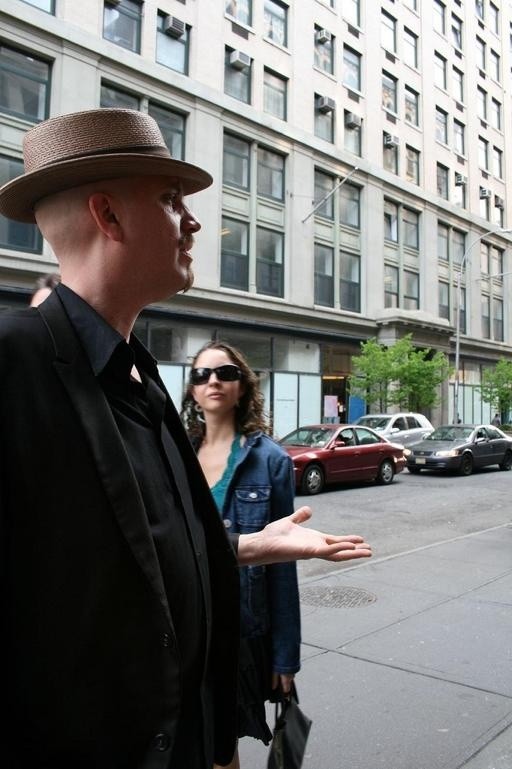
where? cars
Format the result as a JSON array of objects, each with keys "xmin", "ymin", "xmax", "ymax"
[
  {"xmin": 404, "ymin": 423, "xmax": 512, "ymax": 476},
  {"xmin": 353, "ymin": 411, "xmax": 435, "ymax": 449},
  {"xmin": 276, "ymin": 423, "xmax": 407, "ymax": 495}
]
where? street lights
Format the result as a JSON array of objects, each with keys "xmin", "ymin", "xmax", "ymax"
[{"xmin": 453, "ymin": 229, "xmax": 512, "ymax": 425}]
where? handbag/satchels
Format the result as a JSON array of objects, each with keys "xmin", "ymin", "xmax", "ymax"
[{"xmin": 268, "ymin": 696, "xmax": 312, "ymax": 769}]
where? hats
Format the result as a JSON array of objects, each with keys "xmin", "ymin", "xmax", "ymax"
[{"xmin": 0, "ymin": 107, "xmax": 213, "ymax": 224}]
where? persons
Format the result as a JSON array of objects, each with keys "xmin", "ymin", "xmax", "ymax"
[
  {"xmin": 171, "ymin": 337, "xmax": 303, "ymax": 769},
  {"xmin": 0, "ymin": 105, "xmax": 377, "ymax": 767},
  {"xmin": 29, "ymin": 275, "xmax": 62, "ymax": 309},
  {"xmin": 452, "ymin": 412, "xmax": 463, "ymax": 424},
  {"xmin": 491, "ymin": 413, "xmax": 501, "ymax": 427}
]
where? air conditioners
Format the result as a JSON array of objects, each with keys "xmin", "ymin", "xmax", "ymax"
[
  {"xmin": 481, "ymin": 188, "xmax": 491, "ymax": 198},
  {"xmin": 347, "ymin": 113, "xmax": 361, "ymax": 128},
  {"xmin": 230, "ymin": 50, "xmax": 252, "ymax": 70},
  {"xmin": 496, "ymin": 198, "xmax": 503, "ymax": 206},
  {"xmin": 457, "ymin": 175, "xmax": 467, "ymax": 185},
  {"xmin": 318, "ymin": 96, "xmax": 336, "ymax": 111},
  {"xmin": 164, "ymin": 16, "xmax": 185, "ymax": 36},
  {"xmin": 317, "ymin": 30, "xmax": 330, "ymax": 42},
  {"xmin": 386, "ymin": 134, "xmax": 400, "ymax": 148}
]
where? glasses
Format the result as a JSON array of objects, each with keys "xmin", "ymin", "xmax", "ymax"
[{"xmin": 190, "ymin": 365, "xmax": 242, "ymax": 385}]
